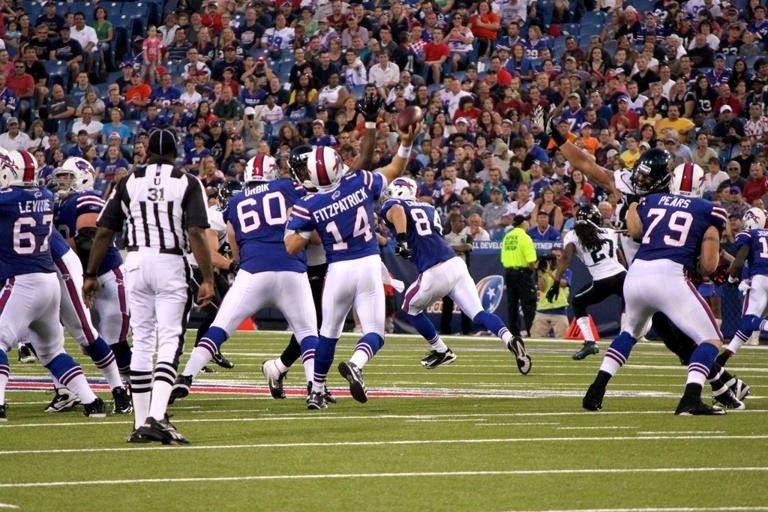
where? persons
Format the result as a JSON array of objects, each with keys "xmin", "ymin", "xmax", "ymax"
[{"xmin": 81, "ymin": 126, "xmax": 216, "ymax": 446}]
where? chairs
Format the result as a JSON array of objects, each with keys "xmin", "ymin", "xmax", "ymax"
[{"xmin": 1, "ymin": 1, "xmax": 767, "ymax": 243}]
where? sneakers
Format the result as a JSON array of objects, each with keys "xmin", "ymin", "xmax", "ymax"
[
  {"xmin": 111, "ymin": 381, "xmax": 133, "ymax": 414},
  {"xmin": 508, "ymin": 335, "xmax": 532, "ymax": 375},
  {"xmin": 43, "ymin": 387, "xmax": 81, "ymax": 413},
  {"xmin": 126, "ymin": 415, "xmax": 193, "ymax": 447},
  {"xmin": 305, "ymin": 381, "xmax": 337, "ymax": 411},
  {"xmin": 210, "ymin": 350, "xmax": 235, "ymax": 369},
  {"xmin": 199, "ymin": 365, "xmax": 218, "ymax": 375},
  {"xmin": 572, "ymin": 342, "xmax": 601, "ymax": 361},
  {"xmin": 260, "ymin": 358, "xmax": 287, "ymax": 399},
  {"xmin": 673, "ymin": 399, "xmax": 729, "ymax": 416},
  {"xmin": 167, "ymin": 372, "xmax": 193, "ymax": 406},
  {"xmin": 582, "ymin": 394, "xmax": 604, "ymax": 411},
  {"xmin": 419, "ymin": 348, "xmax": 457, "ymax": 370},
  {"xmin": 0, "ymin": 403, "xmax": 9, "ymax": 422},
  {"xmin": 709, "ymin": 355, "xmax": 751, "ymax": 413},
  {"xmin": 17, "ymin": 346, "xmax": 36, "ymax": 363},
  {"xmin": 338, "ymin": 360, "xmax": 368, "ymax": 403},
  {"xmin": 80, "ymin": 395, "xmax": 107, "ymax": 418}
]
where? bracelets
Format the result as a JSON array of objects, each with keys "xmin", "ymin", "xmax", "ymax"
[{"xmin": 82, "ymin": 271, "xmax": 97, "ymax": 278}]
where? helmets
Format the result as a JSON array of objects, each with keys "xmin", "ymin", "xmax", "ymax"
[
  {"xmin": 51, "ymin": 156, "xmax": 97, "ymax": 199},
  {"xmin": 667, "ymin": 161, "xmax": 707, "ymax": 200},
  {"xmin": 742, "ymin": 207, "xmax": 767, "ymax": 232},
  {"xmin": 242, "ymin": 152, "xmax": 279, "ymax": 186},
  {"xmin": 630, "ymin": 147, "xmax": 674, "ymax": 197},
  {"xmin": 284, "ymin": 144, "xmax": 320, "ymax": 192},
  {"xmin": 0, "ymin": 146, "xmax": 21, "ymax": 193},
  {"xmin": 380, "ymin": 176, "xmax": 419, "ymax": 202},
  {"xmin": 9, "ymin": 150, "xmax": 40, "ymax": 189},
  {"xmin": 306, "ymin": 145, "xmax": 347, "ymax": 195}
]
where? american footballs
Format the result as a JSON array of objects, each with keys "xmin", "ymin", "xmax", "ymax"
[{"xmin": 397, "ymin": 105, "xmax": 424, "ymax": 132}]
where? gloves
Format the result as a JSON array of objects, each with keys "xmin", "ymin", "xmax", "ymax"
[
  {"xmin": 454, "ymin": 117, "xmax": 469, "ymax": 125},
  {"xmin": 394, "ymin": 241, "xmax": 415, "ymax": 261},
  {"xmin": 354, "ymin": 92, "xmax": 386, "ymax": 130},
  {"xmin": 727, "ymin": 274, "xmax": 739, "ymax": 288},
  {"xmin": 544, "ymin": 280, "xmax": 560, "ymax": 304}
]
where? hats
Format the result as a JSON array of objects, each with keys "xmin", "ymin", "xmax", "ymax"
[
  {"xmin": 147, "ymin": 124, "xmax": 179, "ymax": 161},
  {"xmin": 195, "ymin": 70, "xmax": 208, "ymax": 75},
  {"xmin": 223, "ymin": 45, "xmax": 235, "ymax": 50},
  {"xmin": 317, "ymin": 15, "xmax": 329, "ymax": 23},
  {"xmin": 616, "ymin": 94, "xmax": 629, "ymax": 104},
  {"xmin": 243, "ymin": 106, "xmax": 256, "ymax": 116},
  {"xmin": 346, "ymin": 13, "xmax": 357, "ymax": 20},
  {"xmin": 719, "ymin": 104, "xmax": 733, "ymax": 114},
  {"xmin": 6, "ymin": 116, "xmax": 19, "ymax": 126},
  {"xmin": 493, "ymin": 142, "xmax": 508, "ymax": 157}
]
